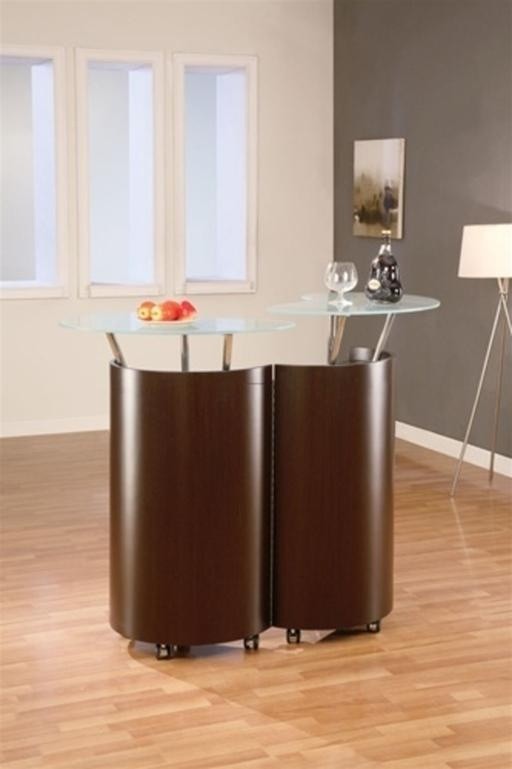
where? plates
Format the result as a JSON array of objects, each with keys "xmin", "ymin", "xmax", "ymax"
[{"xmin": 132, "ymin": 314, "xmax": 195, "ymax": 329}]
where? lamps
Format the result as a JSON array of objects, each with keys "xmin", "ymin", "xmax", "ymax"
[{"xmin": 449, "ymin": 223, "xmax": 512, "ymax": 496}]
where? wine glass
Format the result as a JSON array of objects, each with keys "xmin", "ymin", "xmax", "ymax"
[{"xmin": 326, "ymin": 262, "xmax": 358, "ymax": 306}]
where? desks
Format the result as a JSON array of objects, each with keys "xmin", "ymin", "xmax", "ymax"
[{"xmin": 58, "ymin": 291, "xmax": 441, "ymax": 659}]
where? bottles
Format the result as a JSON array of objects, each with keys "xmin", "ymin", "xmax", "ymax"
[{"xmin": 365, "ymin": 229, "xmax": 403, "ymax": 304}]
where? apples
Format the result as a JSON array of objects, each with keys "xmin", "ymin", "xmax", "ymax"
[{"xmin": 137, "ymin": 299, "xmax": 198, "ymax": 322}]
